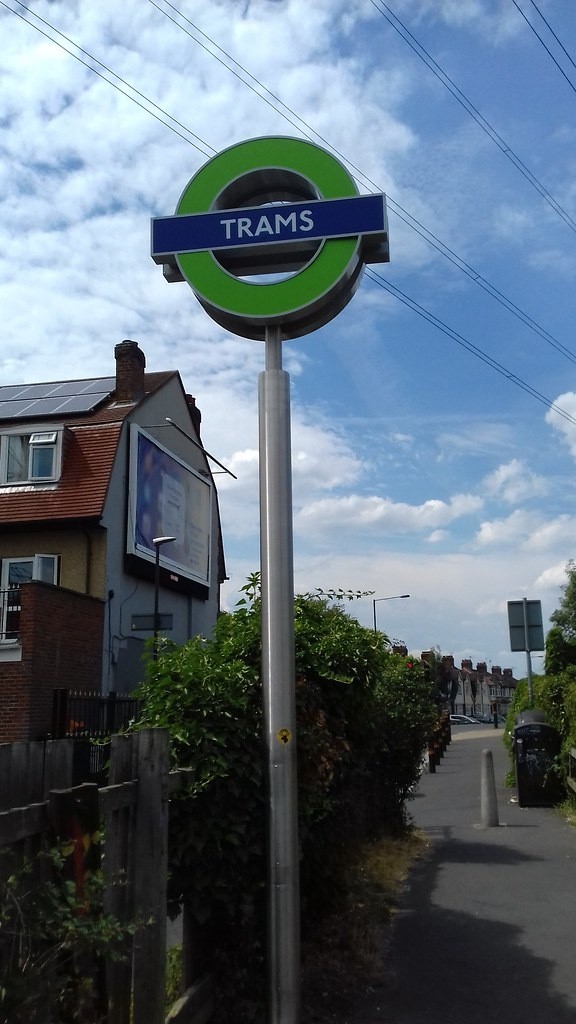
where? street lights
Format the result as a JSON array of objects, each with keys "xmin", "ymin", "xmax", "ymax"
[
  {"xmin": 372, "ymin": 591, "xmax": 410, "ymax": 634},
  {"xmin": 149, "ymin": 535, "xmax": 179, "ymax": 675}
]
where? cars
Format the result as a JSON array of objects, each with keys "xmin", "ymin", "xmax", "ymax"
[{"xmin": 450, "ymin": 715, "xmax": 480, "ymax": 724}]
